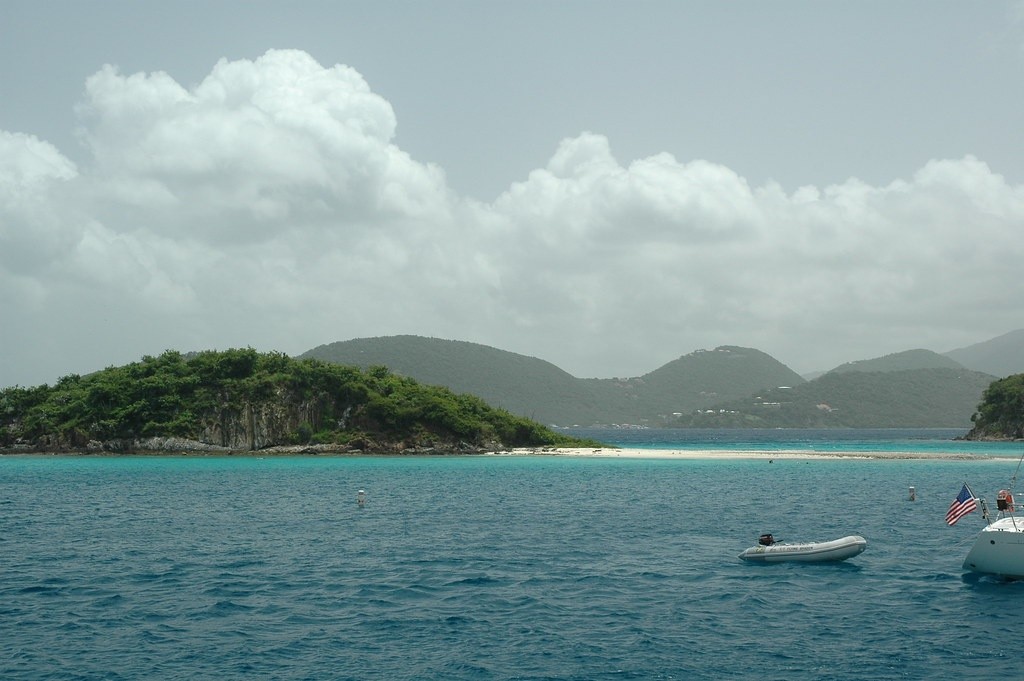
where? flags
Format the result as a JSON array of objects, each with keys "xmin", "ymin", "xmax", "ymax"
[{"xmin": 945, "ymin": 483, "xmax": 977, "ymax": 527}]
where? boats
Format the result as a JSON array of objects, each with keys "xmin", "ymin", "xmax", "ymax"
[
  {"xmin": 961, "ymin": 488, "xmax": 1024, "ymax": 577},
  {"xmin": 736, "ymin": 533, "xmax": 868, "ymax": 562}
]
